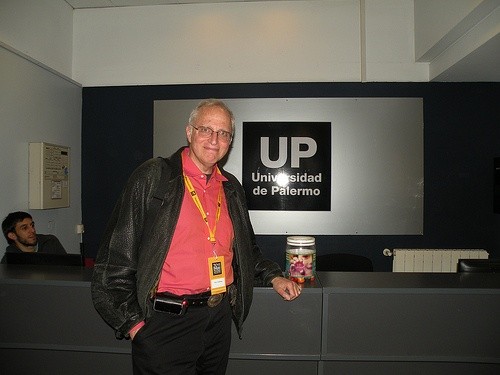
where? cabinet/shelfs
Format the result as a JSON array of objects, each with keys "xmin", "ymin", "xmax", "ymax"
[
  {"xmin": 316, "ymin": 271, "xmax": 500, "ymax": 375},
  {"xmin": 0, "ymin": 268, "xmax": 322, "ymax": 375}
]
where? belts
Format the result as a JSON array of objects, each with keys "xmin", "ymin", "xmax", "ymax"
[{"xmin": 185, "ymin": 286, "xmax": 232, "ymax": 308}]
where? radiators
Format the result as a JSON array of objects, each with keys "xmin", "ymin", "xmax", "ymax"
[{"xmin": 383, "ymin": 248, "xmax": 489, "ymax": 272}]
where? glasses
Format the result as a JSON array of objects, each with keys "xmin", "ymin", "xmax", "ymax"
[{"xmin": 190, "ymin": 123, "xmax": 232, "ymax": 142}]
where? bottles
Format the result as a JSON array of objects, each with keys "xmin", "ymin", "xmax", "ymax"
[{"xmin": 285, "ymin": 235, "xmax": 316, "ymax": 283}]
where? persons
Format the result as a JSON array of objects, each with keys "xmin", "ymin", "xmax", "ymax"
[
  {"xmin": 1, "ymin": 211, "xmax": 67, "ymax": 264},
  {"xmin": 91, "ymin": 98, "xmax": 302, "ymax": 375}
]
